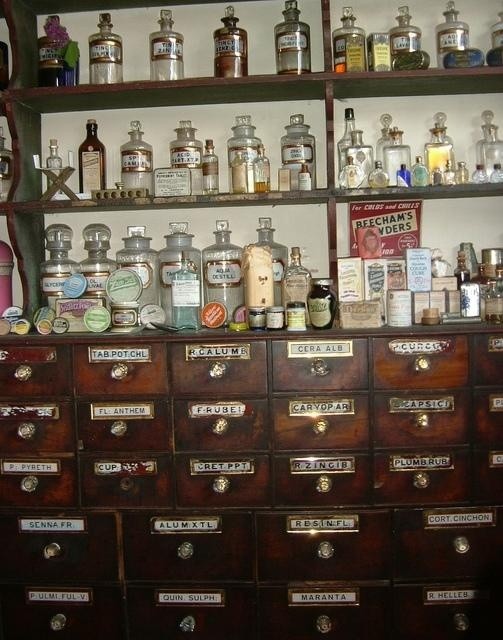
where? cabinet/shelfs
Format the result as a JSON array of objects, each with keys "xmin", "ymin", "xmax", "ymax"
[{"xmin": 0, "ymin": 0, "xmax": 502, "ymax": 640}]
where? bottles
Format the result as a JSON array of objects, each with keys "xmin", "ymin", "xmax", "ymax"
[
  {"xmin": 337, "ymin": 108, "xmax": 501, "ymax": 193},
  {"xmin": 1, "ymin": 223, "xmax": 337, "ymax": 333},
  {"xmin": 334, "ymin": 0, "xmax": 503, "ymax": 73},
  {"xmin": 34, "ymin": 2, "xmax": 311, "ymax": 91},
  {"xmin": 1, "ymin": 112, "xmax": 319, "ymax": 200},
  {"xmin": 457, "ymin": 242, "xmax": 503, "ymax": 328}
]
